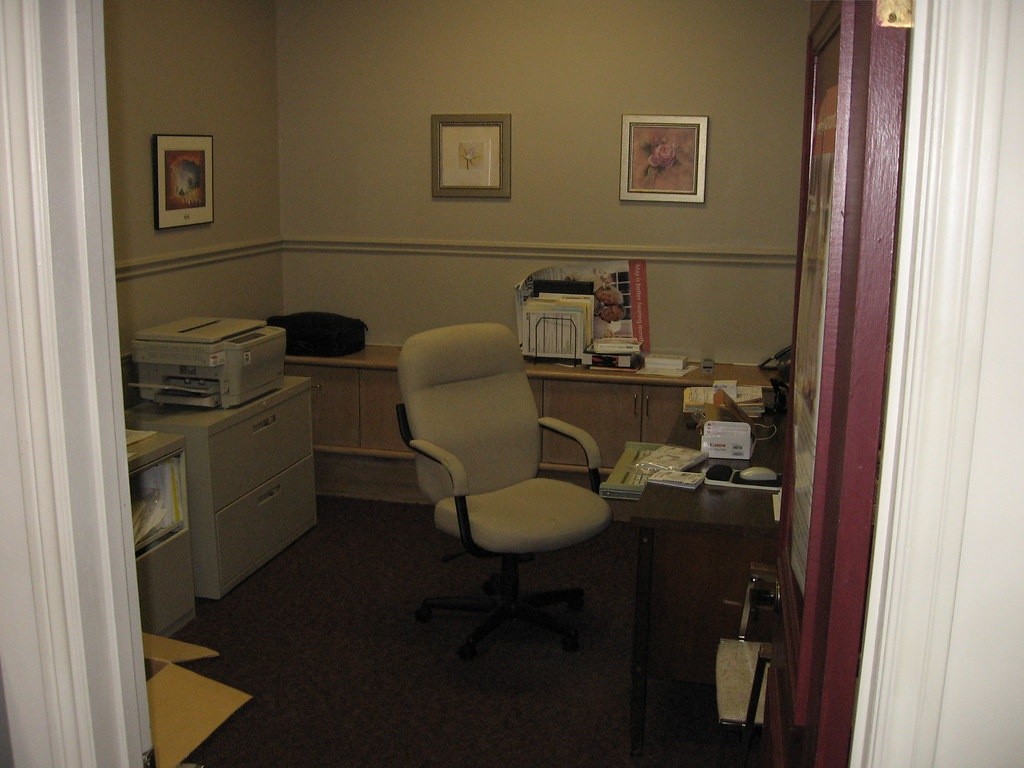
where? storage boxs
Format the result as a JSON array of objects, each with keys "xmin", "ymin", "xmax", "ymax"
[{"xmin": 140, "ymin": 630, "xmax": 255, "ymax": 768}]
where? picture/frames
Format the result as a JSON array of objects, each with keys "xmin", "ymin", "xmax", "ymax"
[
  {"xmin": 619, "ymin": 113, "xmax": 710, "ymax": 204},
  {"xmin": 152, "ymin": 133, "xmax": 216, "ymax": 230},
  {"xmin": 430, "ymin": 112, "xmax": 512, "ymax": 198}
]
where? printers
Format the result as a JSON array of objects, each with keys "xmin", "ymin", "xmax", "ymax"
[{"xmin": 126, "ymin": 316, "xmax": 287, "ymax": 409}]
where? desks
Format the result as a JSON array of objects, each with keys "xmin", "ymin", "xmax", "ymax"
[{"xmin": 627, "ymin": 386, "xmax": 782, "ymax": 759}]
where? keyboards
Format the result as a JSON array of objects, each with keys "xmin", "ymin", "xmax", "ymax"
[{"xmin": 625, "ymin": 449, "xmax": 654, "ymax": 485}]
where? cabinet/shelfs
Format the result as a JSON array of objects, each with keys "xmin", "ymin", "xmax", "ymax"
[{"xmin": 285, "ymin": 345, "xmax": 789, "ymax": 530}]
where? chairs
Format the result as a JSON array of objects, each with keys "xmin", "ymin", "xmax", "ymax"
[{"xmin": 393, "ymin": 322, "xmax": 616, "ymax": 663}]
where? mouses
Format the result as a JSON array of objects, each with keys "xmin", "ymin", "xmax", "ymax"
[{"xmin": 739, "ymin": 466, "xmax": 777, "ymax": 481}]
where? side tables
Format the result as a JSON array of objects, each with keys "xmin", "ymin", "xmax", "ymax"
[
  {"xmin": 121, "ymin": 376, "xmax": 320, "ymax": 602},
  {"xmin": 124, "ymin": 429, "xmax": 196, "ymax": 640}
]
